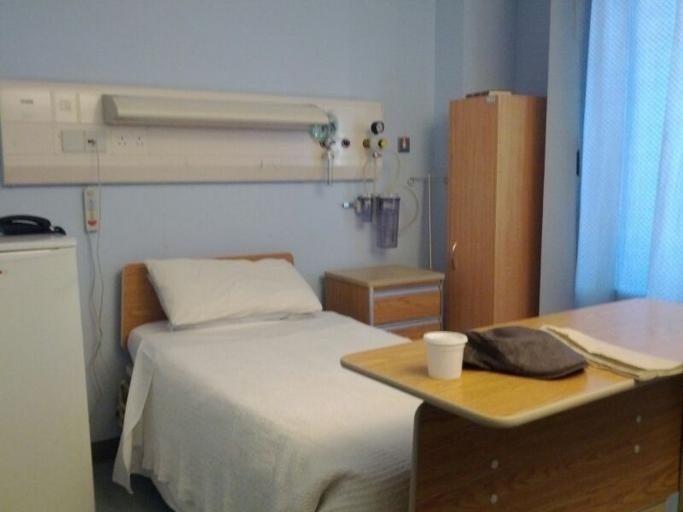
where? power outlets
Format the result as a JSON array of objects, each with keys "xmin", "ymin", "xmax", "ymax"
[{"xmin": 84, "ymin": 129, "xmax": 146, "ymax": 153}]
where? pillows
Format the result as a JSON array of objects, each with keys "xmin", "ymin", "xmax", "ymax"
[{"xmin": 143, "ymin": 257, "xmax": 323, "ymax": 330}]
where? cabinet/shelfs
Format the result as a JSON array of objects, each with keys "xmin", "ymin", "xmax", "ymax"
[
  {"xmin": 324, "ymin": 264, "xmax": 445, "ymax": 342},
  {"xmin": 445, "ymin": 96, "xmax": 546, "ymax": 333}
]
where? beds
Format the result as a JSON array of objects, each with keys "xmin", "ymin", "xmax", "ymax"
[{"xmin": 119, "ymin": 251, "xmax": 424, "ymax": 512}]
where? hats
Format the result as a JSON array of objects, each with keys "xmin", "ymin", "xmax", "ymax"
[{"xmin": 461, "ymin": 327, "xmax": 588, "ymax": 379}]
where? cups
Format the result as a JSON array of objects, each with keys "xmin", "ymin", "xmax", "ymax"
[{"xmin": 423, "ymin": 331, "xmax": 468, "ymax": 380}]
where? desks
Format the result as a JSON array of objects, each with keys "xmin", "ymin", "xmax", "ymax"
[{"xmin": 339, "ymin": 297, "xmax": 683, "ymax": 512}]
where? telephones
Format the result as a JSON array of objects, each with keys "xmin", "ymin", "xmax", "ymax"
[{"xmin": 0, "ymin": 215, "xmax": 51, "ymax": 234}]
